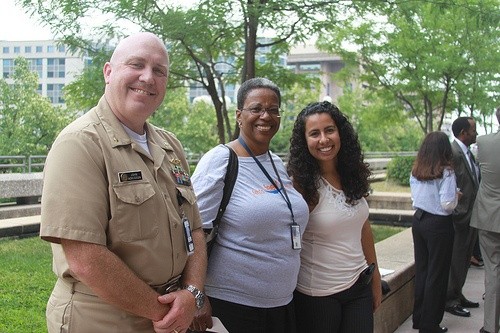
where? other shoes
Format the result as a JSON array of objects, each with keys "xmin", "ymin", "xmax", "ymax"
[
  {"xmin": 446, "ymin": 303, "xmax": 470, "ymax": 316},
  {"xmin": 412, "ymin": 324, "xmax": 448, "ymax": 333},
  {"xmin": 462, "ymin": 300, "xmax": 479, "ymax": 308},
  {"xmin": 471, "ymin": 261, "xmax": 485, "ymax": 267},
  {"xmin": 480, "ymin": 326, "xmax": 490, "ymax": 333}
]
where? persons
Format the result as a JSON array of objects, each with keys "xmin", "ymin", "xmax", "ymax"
[
  {"xmin": 285, "ymin": 101, "xmax": 382, "ymax": 333},
  {"xmin": 39, "ymin": 32, "xmax": 207, "ymax": 333},
  {"xmin": 190, "ymin": 77, "xmax": 309, "ymax": 333},
  {"xmin": 409, "ymin": 105, "xmax": 500, "ymax": 333}
]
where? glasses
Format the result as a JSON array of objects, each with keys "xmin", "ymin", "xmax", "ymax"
[{"xmin": 242, "ymin": 103, "xmax": 285, "ymax": 117}]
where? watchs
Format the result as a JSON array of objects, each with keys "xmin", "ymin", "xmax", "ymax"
[{"xmin": 181, "ymin": 284, "xmax": 205, "ymax": 310}]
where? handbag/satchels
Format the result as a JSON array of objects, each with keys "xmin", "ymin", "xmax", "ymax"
[{"xmin": 203, "ymin": 228, "xmax": 217, "ymax": 258}]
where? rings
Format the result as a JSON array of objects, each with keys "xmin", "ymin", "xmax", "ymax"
[{"xmin": 174, "ymin": 330, "xmax": 178, "ymax": 333}]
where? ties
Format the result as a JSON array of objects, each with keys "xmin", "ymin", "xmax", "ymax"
[{"xmin": 469, "ymin": 150, "xmax": 479, "ymax": 189}]
